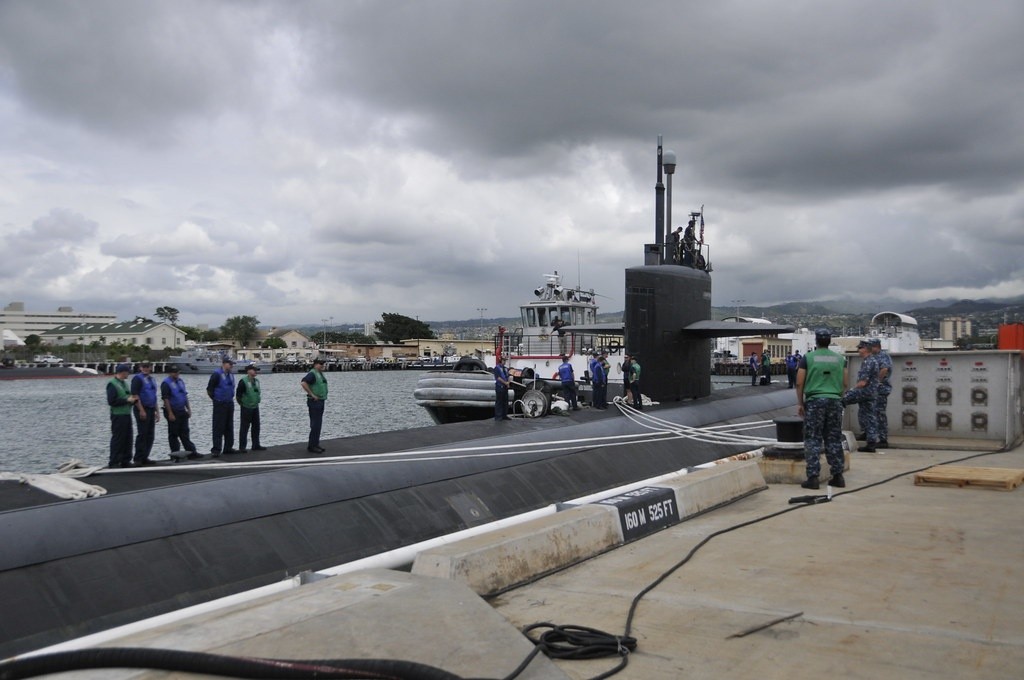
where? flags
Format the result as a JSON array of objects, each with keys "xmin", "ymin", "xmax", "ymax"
[{"xmin": 700, "ymin": 207, "xmax": 704, "ymax": 244}]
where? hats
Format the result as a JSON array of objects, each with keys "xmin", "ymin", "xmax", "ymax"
[
  {"xmin": 222, "ymin": 357, "xmax": 237, "ymax": 365},
  {"xmin": 314, "ymin": 357, "xmax": 327, "ymax": 365},
  {"xmin": 168, "ymin": 365, "xmax": 181, "ymax": 373},
  {"xmin": 856, "ymin": 340, "xmax": 873, "ymax": 347},
  {"xmin": 870, "ymin": 338, "xmax": 881, "ymax": 345},
  {"xmin": 815, "ymin": 328, "xmax": 832, "ymax": 339},
  {"xmin": 117, "ymin": 365, "xmax": 132, "ymax": 372},
  {"xmin": 498, "ymin": 357, "xmax": 508, "ymax": 361},
  {"xmin": 142, "ymin": 360, "xmax": 151, "ymax": 366},
  {"xmin": 247, "ymin": 364, "xmax": 260, "ymax": 371}
]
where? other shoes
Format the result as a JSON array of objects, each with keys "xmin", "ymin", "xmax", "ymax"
[
  {"xmin": 109, "ymin": 464, "xmax": 120, "ymax": 468},
  {"xmin": 309, "ymin": 447, "xmax": 322, "ymax": 453},
  {"xmin": 212, "ymin": 450, "xmax": 222, "ymax": 456},
  {"xmin": 317, "ymin": 445, "xmax": 325, "ymax": 451},
  {"xmin": 135, "ymin": 460, "xmax": 144, "ymax": 467},
  {"xmin": 223, "ymin": 450, "xmax": 240, "ymax": 454},
  {"xmin": 252, "ymin": 446, "xmax": 267, "ymax": 450},
  {"xmin": 121, "ymin": 462, "xmax": 135, "ymax": 468},
  {"xmin": 503, "ymin": 416, "xmax": 512, "ymax": 420},
  {"xmin": 143, "ymin": 459, "xmax": 155, "ymax": 464},
  {"xmin": 189, "ymin": 451, "xmax": 204, "ymax": 458},
  {"xmin": 240, "ymin": 448, "xmax": 247, "ymax": 453}
]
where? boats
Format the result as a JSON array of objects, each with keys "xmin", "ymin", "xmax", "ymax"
[
  {"xmin": 414, "ymin": 250, "xmax": 625, "ymax": 424},
  {"xmin": 169, "ymin": 334, "xmax": 276, "ymax": 374}
]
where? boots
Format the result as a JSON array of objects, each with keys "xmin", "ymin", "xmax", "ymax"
[
  {"xmin": 802, "ymin": 477, "xmax": 819, "ymax": 488},
  {"xmin": 856, "ymin": 431, "xmax": 867, "ymax": 440},
  {"xmin": 828, "ymin": 474, "xmax": 845, "ymax": 487},
  {"xmin": 875, "ymin": 439, "xmax": 888, "ymax": 448},
  {"xmin": 858, "ymin": 442, "xmax": 876, "ymax": 452}
]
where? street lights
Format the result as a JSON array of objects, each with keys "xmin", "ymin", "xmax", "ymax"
[
  {"xmin": 81, "ymin": 323, "xmax": 87, "ymax": 361},
  {"xmin": 477, "ymin": 308, "xmax": 488, "ymax": 362},
  {"xmin": 321, "ymin": 319, "xmax": 328, "ymax": 358}
]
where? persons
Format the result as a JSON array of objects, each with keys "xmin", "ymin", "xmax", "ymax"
[
  {"xmin": 236, "ymin": 364, "xmax": 267, "ymax": 453},
  {"xmin": 621, "ymin": 355, "xmax": 631, "ymax": 399},
  {"xmin": 671, "ymin": 227, "xmax": 682, "ymax": 263},
  {"xmin": 494, "ymin": 355, "xmax": 513, "ymax": 421},
  {"xmin": 684, "ymin": 220, "xmax": 700, "ymax": 266},
  {"xmin": 787, "ymin": 347, "xmax": 818, "ymax": 389},
  {"xmin": 206, "ymin": 355, "xmax": 242, "ymax": 456},
  {"xmin": 550, "ymin": 316, "xmax": 558, "ymax": 326},
  {"xmin": 558, "ymin": 356, "xmax": 580, "ymax": 410},
  {"xmin": 750, "ymin": 352, "xmax": 759, "ymax": 386},
  {"xmin": 550, "ymin": 320, "xmax": 567, "ymax": 356},
  {"xmin": 131, "ymin": 359, "xmax": 160, "ymax": 465},
  {"xmin": 842, "ymin": 339, "xmax": 880, "ymax": 453},
  {"xmin": 593, "ymin": 357, "xmax": 606, "ymax": 410},
  {"xmin": 716, "ymin": 350, "xmax": 733, "ymax": 358},
  {"xmin": 760, "ymin": 350, "xmax": 772, "ymax": 386},
  {"xmin": 597, "ymin": 351, "xmax": 610, "ymax": 406},
  {"xmin": 590, "ymin": 352, "xmax": 608, "ymax": 409},
  {"xmin": 106, "ymin": 364, "xmax": 139, "ymax": 468},
  {"xmin": 628, "ymin": 356, "xmax": 642, "ymax": 410},
  {"xmin": 796, "ymin": 328, "xmax": 848, "ymax": 490},
  {"xmin": 161, "ymin": 365, "xmax": 204, "ymax": 461},
  {"xmin": 855, "ymin": 338, "xmax": 893, "ymax": 448},
  {"xmin": 301, "ymin": 357, "xmax": 328, "ymax": 454}
]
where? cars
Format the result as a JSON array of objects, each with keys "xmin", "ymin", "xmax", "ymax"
[
  {"xmin": 396, "ymin": 355, "xmax": 408, "ymax": 362},
  {"xmin": 34, "ymin": 354, "xmax": 64, "ymax": 363},
  {"xmin": 421, "ymin": 356, "xmax": 430, "ymax": 362},
  {"xmin": 287, "ymin": 355, "xmax": 385, "ymax": 363}
]
